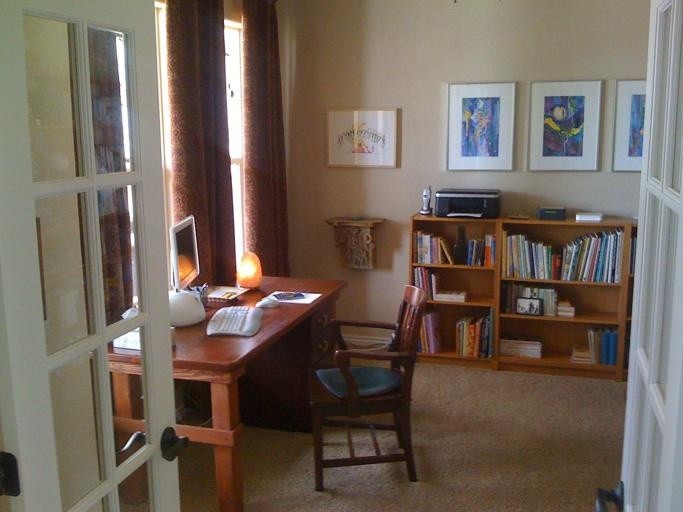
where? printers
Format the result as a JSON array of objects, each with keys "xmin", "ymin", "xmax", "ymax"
[{"xmin": 435, "ymin": 187, "xmax": 502, "ymax": 219}]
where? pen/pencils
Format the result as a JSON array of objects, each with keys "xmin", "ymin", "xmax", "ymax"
[{"xmin": 187, "ymin": 282, "xmax": 208, "ymax": 292}]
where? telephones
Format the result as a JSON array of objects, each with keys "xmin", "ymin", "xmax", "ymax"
[{"xmin": 420, "ymin": 184, "xmax": 433, "ymax": 215}]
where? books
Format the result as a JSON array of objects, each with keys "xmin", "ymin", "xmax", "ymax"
[{"xmin": 413, "ymin": 227, "xmax": 624, "ymax": 365}]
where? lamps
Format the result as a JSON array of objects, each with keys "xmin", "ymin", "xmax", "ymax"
[{"xmin": 237, "ymin": 251, "xmax": 262, "ymax": 290}]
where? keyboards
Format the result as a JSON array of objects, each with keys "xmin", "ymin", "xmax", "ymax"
[{"xmin": 206, "ymin": 305, "xmax": 265, "ymax": 336}]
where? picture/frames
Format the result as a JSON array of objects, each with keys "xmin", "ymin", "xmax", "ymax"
[
  {"xmin": 527, "ymin": 78, "xmax": 603, "ymax": 172},
  {"xmin": 325, "ymin": 107, "xmax": 398, "ymax": 169},
  {"xmin": 446, "ymin": 81, "xmax": 516, "ymax": 170},
  {"xmin": 611, "ymin": 79, "xmax": 646, "ymax": 173}
]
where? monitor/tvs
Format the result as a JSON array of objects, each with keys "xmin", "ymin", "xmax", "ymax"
[{"xmin": 168, "ymin": 215, "xmax": 205, "ymax": 328}]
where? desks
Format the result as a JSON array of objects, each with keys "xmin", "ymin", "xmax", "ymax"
[{"xmin": 107, "ymin": 275, "xmax": 348, "ymax": 512}]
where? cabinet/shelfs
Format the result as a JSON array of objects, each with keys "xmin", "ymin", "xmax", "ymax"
[{"xmin": 409, "ymin": 211, "xmax": 637, "ymax": 380}]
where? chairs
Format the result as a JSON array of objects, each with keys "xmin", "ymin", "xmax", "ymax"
[{"xmin": 310, "ymin": 284, "xmax": 427, "ymax": 490}]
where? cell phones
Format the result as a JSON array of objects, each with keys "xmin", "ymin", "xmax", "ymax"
[{"xmin": 273, "ymin": 292, "xmax": 304, "ymax": 300}]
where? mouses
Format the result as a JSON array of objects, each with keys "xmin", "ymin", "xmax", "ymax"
[{"xmin": 254, "ymin": 298, "xmax": 279, "ymax": 309}]
